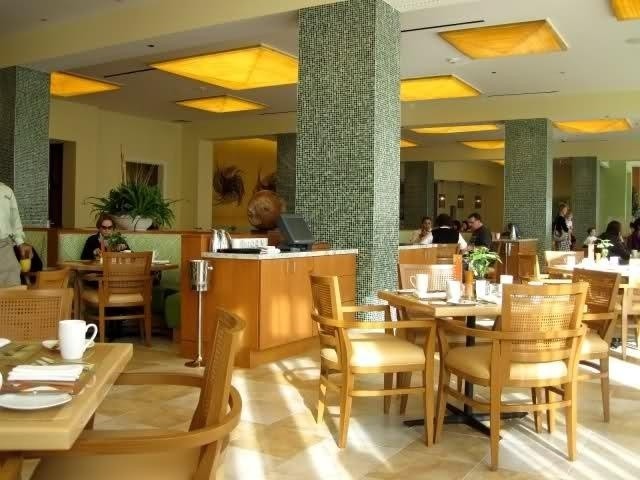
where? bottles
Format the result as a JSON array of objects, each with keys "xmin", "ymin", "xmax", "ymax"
[
  {"xmin": 510, "ymin": 224, "xmax": 517, "ymax": 241},
  {"xmin": 465, "ymin": 271, "xmax": 474, "ymax": 300}
]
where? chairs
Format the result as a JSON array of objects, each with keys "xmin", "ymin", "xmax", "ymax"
[
  {"xmin": 309, "ymin": 273, "xmax": 439, "ymax": 450},
  {"xmin": 77, "ymin": 251, "xmax": 153, "ymax": 344},
  {"xmin": 1, "ymin": 286, "xmax": 74, "ymax": 479},
  {"xmin": 34, "ymin": 267, "xmax": 71, "ymax": 289},
  {"xmin": 437, "ymin": 280, "xmax": 589, "ymax": 469},
  {"xmin": 31, "ymin": 308, "xmax": 247, "ymax": 479},
  {"xmin": 394, "ymin": 253, "xmax": 466, "ymax": 415},
  {"xmin": 530, "ymin": 267, "xmax": 621, "ymax": 436},
  {"xmin": 400, "ymin": 236, "xmax": 639, "ymax": 353}
]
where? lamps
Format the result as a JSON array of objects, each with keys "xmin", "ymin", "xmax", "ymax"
[{"xmin": 438, "ymin": 181, "xmax": 482, "ymax": 209}]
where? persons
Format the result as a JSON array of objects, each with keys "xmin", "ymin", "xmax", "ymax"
[
  {"xmin": 410, "ymin": 213, "xmax": 492, "ymax": 251},
  {"xmin": 553, "ymin": 201, "xmax": 640, "ymax": 259},
  {"xmin": 81, "ymin": 214, "xmax": 129, "ymax": 286},
  {"xmin": 0, "ymin": 177, "xmax": 32, "ymax": 288},
  {"xmin": 18, "ymin": 244, "xmax": 43, "ymax": 284}
]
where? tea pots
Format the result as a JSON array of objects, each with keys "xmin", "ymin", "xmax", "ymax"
[{"xmin": 208, "ymin": 228, "xmax": 232, "ymax": 253}]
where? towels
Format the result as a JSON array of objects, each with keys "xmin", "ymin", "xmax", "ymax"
[{"xmin": 8, "ymin": 362, "xmax": 85, "ymax": 382}]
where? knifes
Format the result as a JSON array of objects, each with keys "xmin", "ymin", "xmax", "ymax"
[{"xmin": 11, "ymin": 389, "xmax": 72, "ymax": 395}]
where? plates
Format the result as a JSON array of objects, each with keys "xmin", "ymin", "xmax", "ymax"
[
  {"xmin": 448, "ymin": 298, "xmax": 476, "ymax": 305},
  {"xmin": 0, "ymin": 385, "xmax": 73, "ymax": 411},
  {"xmin": 42, "ymin": 338, "xmax": 95, "ymax": 350},
  {"xmin": 153, "ymin": 260, "xmax": 170, "ymax": 265}
]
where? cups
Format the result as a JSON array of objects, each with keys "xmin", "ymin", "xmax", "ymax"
[
  {"xmin": 499, "ymin": 274, "xmax": 513, "ymax": 297},
  {"xmin": 265, "ymin": 245, "xmax": 275, "ymax": 255},
  {"xmin": 565, "ymin": 254, "xmax": 618, "ymax": 270},
  {"xmin": 445, "ymin": 280, "xmax": 460, "ymax": 299},
  {"xmin": 496, "ymin": 233, "xmax": 500, "ymax": 240},
  {"xmin": 19, "ymin": 243, "xmax": 33, "ymax": 274},
  {"xmin": 58, "ymin": 320, "xmax": 103, "ymax": 360},
  {"xmin": 410, "ymin": 273, "xmax": 429, "ymax": 293}
]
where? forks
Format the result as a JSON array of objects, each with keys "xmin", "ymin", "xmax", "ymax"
[{"xmin": 1, "ymin": 345, "xmax": 26, "ymax": 357}]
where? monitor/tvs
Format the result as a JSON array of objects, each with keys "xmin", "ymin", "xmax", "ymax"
[{"xmin": 281, "ymin": 214, "xmax": 316, "ymax": 245}]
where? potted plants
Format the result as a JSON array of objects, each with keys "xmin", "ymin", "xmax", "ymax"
[{"xmin": 84, "ymin": 177, "xmax": 177, "ymax": 231}]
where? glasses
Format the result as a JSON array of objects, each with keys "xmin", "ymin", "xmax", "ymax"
[{"xmin": 100, "ymin": 225, "xmax": 114, "ymax": 230}]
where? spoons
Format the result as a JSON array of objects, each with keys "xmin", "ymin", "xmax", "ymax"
[{"xmin": 36, "ymin": 356, "xmax": 90, "ymax": 366}]
